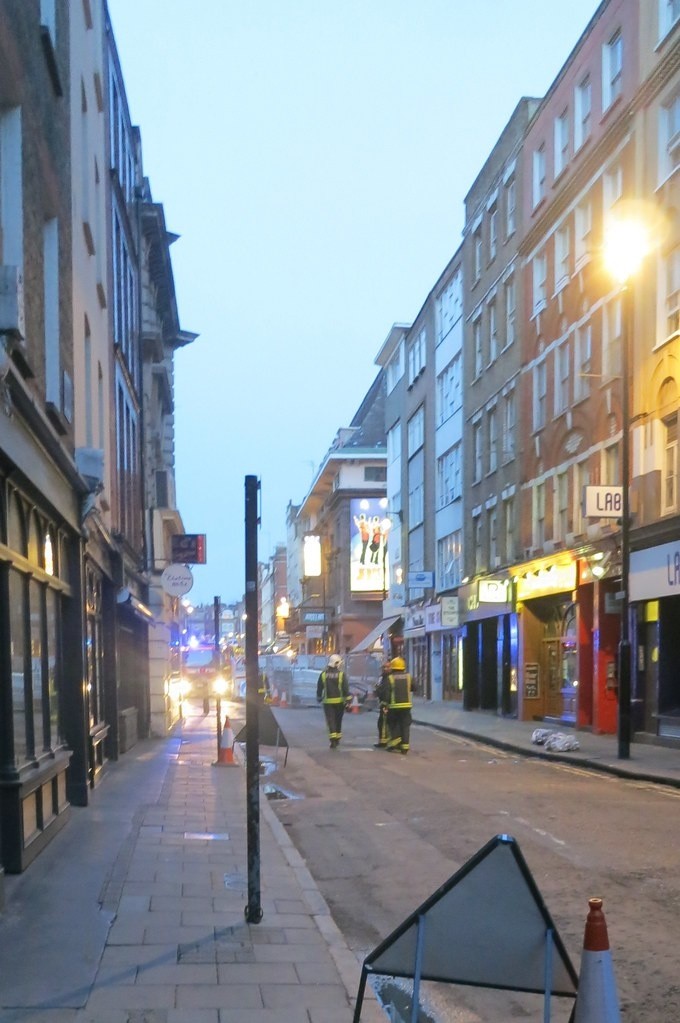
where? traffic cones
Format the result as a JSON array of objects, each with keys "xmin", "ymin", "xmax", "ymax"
[
  {"xmin": 271, "ymin": 685, "xmax": 280, "ymax": 707},
  {"xmin": 351, "ymin": 693, "xmax": 362, "ymax": 714},
  {"xmin": 212, "ymin": 716, "xmax": 239, "ymax": 768},
  {"xmin": 279, "ymin": 689, "xmax": 288, "ymax": 709},
  {"xmin": 569, "ymin": 897, "xmax": 620, "ymax": 1022}
]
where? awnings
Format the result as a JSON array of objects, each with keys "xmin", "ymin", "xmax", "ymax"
[{"xmin": 352, "ymin": 616, "xmax": 400, "ymax": 653}]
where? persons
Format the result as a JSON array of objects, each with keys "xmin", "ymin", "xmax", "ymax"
[
  {"xmin": 372, "ymin": 657, "xmax": 416, "ymax": 755},
  {"xmin": 354, "ymin": 513, "xmax": 390, "ymax": 564},
  {"xmin": 258, "ymin": 668, "xmax": 272, "ymax": 703},
  {"xmin": 317, "ymin": 654, "xmax": 353, "ymax": 748}
]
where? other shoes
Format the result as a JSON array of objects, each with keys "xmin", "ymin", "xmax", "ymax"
[
  {"xmin": 373, "ymin": 743, "xmax": 387, "ymax": 748},
  {"xmin": 401, "ymin": 749, "xmax": 408, "ymax": 755},
  {"xmin": 330, "ymin": 739, "xmax": 339, "ymax": 748},
  {"xmin": 385, "ymin": 746, "xmax": 398, "ymax": 751}
]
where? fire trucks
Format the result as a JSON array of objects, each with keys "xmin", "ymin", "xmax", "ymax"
[{"xmin": 180, "ymin": 645, "xmax": 228, "ymax": 698}]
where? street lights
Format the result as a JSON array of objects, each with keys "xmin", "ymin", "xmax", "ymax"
[{"xmin": 609, "ymin": 200, "xmax": 650, "ymax": 763}]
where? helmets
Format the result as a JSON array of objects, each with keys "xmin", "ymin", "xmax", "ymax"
[
  {"xmin": 388, "ymin": 657, "xmax": 406, "ymax": 670},
  {"xmin": 327, "ymin": 654, "xmax": 342, "ymax": 669},
  {"xmin": 380, "ymin": 663, "xmax": 392, "ymax": 675}
]
